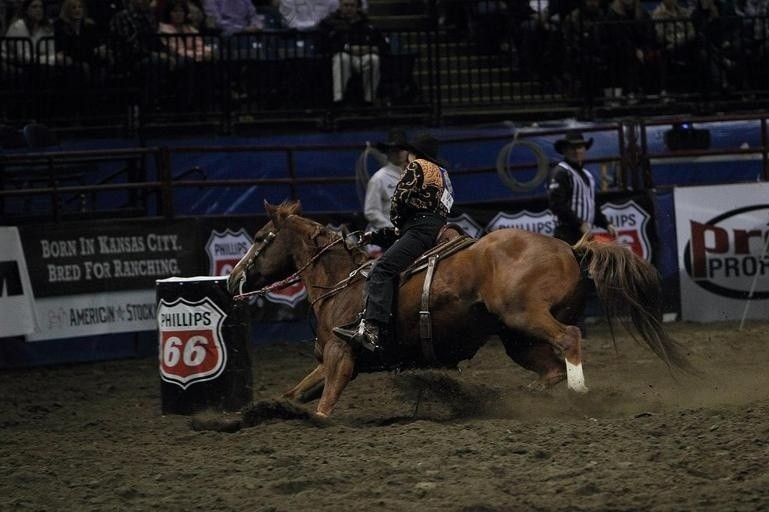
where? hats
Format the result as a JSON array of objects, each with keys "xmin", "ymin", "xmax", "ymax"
[
  {"xmin": 402, "ymin": 132, "xmax": 447, "ymax": 166},
  {"xmin": 553, "ymin": 131, "xmax": 595, "ymax": 153}
]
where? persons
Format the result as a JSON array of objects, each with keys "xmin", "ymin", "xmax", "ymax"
[
  {"xmin": 330, "ymin": 133, "xmax": 454, "ymax": 353},
  {"xmin": 544, "ymin": 129, "xmax": 597, "ymax": 337},
  {"xmin": 363, "ymin": 128, "xmax": 414, "ymax": 242}
]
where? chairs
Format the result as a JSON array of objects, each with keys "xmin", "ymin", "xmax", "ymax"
[{"xmin": 0, "ymin": 0, "xmax": 769, "ymax": 111}]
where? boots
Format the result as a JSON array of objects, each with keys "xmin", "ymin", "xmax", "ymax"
[{"xmin": 333, "ymin": 320, "xmax": 382, "ymax": 352}]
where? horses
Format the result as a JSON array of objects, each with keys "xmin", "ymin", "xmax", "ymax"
[{"xmin": 227, "ymin": 199, "xmax": 715, "ymax": 419}]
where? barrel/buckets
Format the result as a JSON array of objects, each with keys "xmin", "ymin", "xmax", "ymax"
[{"xmin": 155, "ymin": 275, "xmax": 252, "ymax": 416}]
